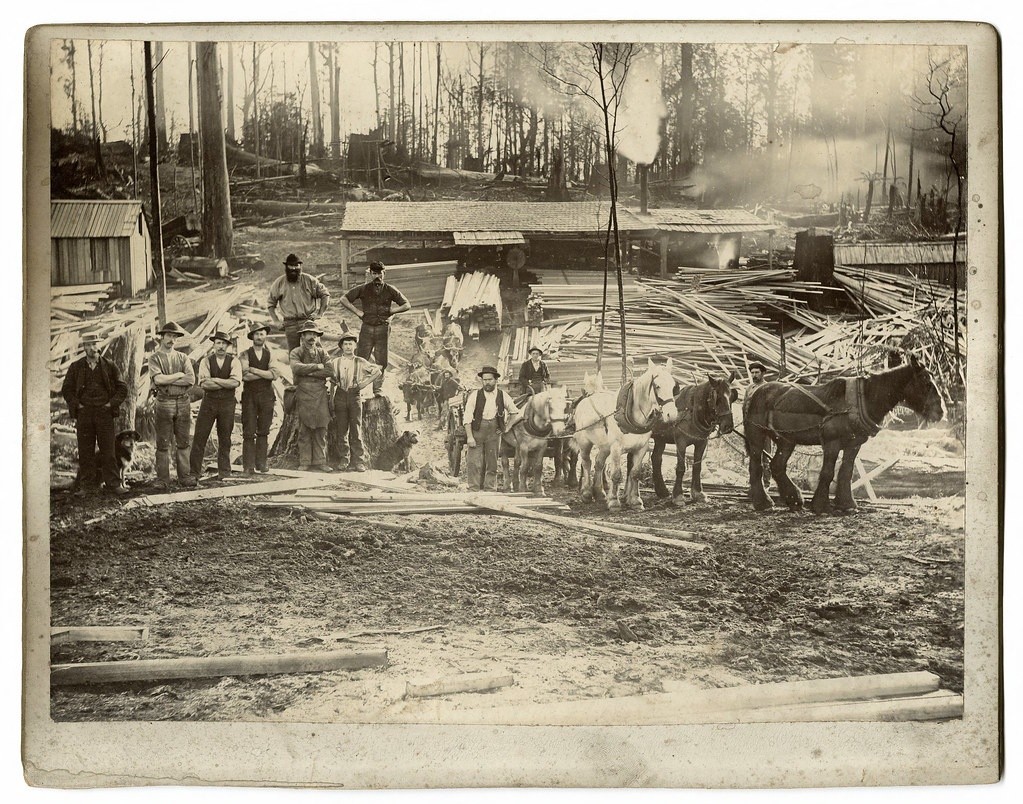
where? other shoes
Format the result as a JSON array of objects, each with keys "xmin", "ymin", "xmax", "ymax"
[
  {"xmin": 179, "ymin": 477, "xmax": 196, "ymax": 486},
  {"xmin": 107, "ymin": 486, "xmax": 129, "ymax": 494},
  {"xmin": 317, "ymin": 464, "xmax": 333, "ymax": 473},
  {"xmin": 152, "ymin": 481, "xmax": 171, "ymax": 489},
  {"xmin": 241, "ymin": 467, "xmax": 255, "ymax": 477},
  {"xmin": 75, "ymin": 489, "xmax": 91, "ymax": 498},
  {"xmin": 298, "ymin": 465, "xmax": 309, "ymax": 471},
  {"xmin": 355, "ymin": 463, "xmax": 366, "ymax": 472},
  {"xmin": 191, "ymin": 475, "xmax": 198, "ymax": 481},
  {"xmin": 256, "ymin": 464, "xmax": 270, "ymax": 471},
  {"xmin": 217, "ymin": 474, "xmax": 238, "ymax": 481},
  {"xmin": 337, "ymin": 464, "xmax": 347, "ymax": 470}
]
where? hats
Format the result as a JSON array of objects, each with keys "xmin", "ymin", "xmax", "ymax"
[
  {"xmin": 338, "ymin": 332, "xmax": 357, "ymax": 347},
  {"xmin": 282, "ymin": 253, "xmax": 304, "ymax": 265},
  {"xmin": 370, "ymin": 261, "xmax": 385, "ymax": 272},
  {"xmin": 748, "ymin": 361, "xmax": 767, "ymax": 373},
  {"xmin": 477, "ymin": 367, "xmax": 502, "ymax": 377},
  {"xmin": 528, "ymin": 346, "xmax": 542, "ymax": 356},
  {"xmin": 157, "ymin": 322, "xmax": 184, "ymax": 337},
  {"xmin": 247, "ymin": 323, "xmax": 271, "ymax": 340},
  {"xmin": 296, "ymin": 320, "xmax": 323, "ymax": 337},
  {"xmin": 210, "ymin": 332, "xmax": 233, "ymax": 345},
  {"xmin": 81, "ymin": 332, "xmax": 104, "ymax": 343}
]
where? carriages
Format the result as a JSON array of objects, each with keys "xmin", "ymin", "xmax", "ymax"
[
  {"xmin": 442, "ymin": 353, "xmax": 949, "ymax": 513},
  {"xmin": 398, "ymin": 364, "xmax": 469, "ymax": 422}
]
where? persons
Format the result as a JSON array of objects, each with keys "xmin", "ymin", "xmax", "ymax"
[
  {"xmin": 341, "ymin": 261, "xmax": 411, "ymax": 398},
  {"xmin": 520, "ymin": 346, "xmax": 551, "ymax": 393},
  {"xmin": 190, "ymin": 332, "xmax": 242, "ymax": 482},
  {"xmin": 238, "ymin": 323, "xmax": 280, "ymax": 477},
  {"xmin": 744, "ymin": 361, "xmax": 771, "ymax": 491},
  {"xmin": 61, "ymin": 333, "xmax": 129, "ymax": 497},
  {"xmin": 148, "ymin": 323, "xmax": 197, "ymax": 489},
  {"xmin": 329, "ymin": 334, "xmax": 383, "ymax": 472},
  {"xmin": 463, "ymin": 366, "xmax": 518, "ymax": 493},
  {"xmin": 289, "ymin": 322, "xmax": 333, "ymax": 471},
  {"xmin": 267, "ymin": 254, "xmax": 331, "ymax": 349}
]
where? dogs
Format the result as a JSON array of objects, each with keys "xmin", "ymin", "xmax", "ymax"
[
  {"xmin": 75, "ymin": 431, "xmax": 141, "ymax": 491},
  {"xmin": 376, "ymin": 430, "xmax": 420, "ymax": 473}
]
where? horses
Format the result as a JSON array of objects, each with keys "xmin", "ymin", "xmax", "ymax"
[
  {"xmin": 500, "ymin": 383, "xmax": 568, "ymax": 498},
  {"xmin": 742, "ymin": 353, "xmax": 944, "ymax": 514},
  {"xmin": 573, "ymin": 355, "xmax": 739, "ymax": 512}
]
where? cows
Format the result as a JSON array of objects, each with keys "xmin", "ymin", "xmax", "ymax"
[{"xmin": 398, "ymin": 322, "xmax": 464, "ymax": 422}]
